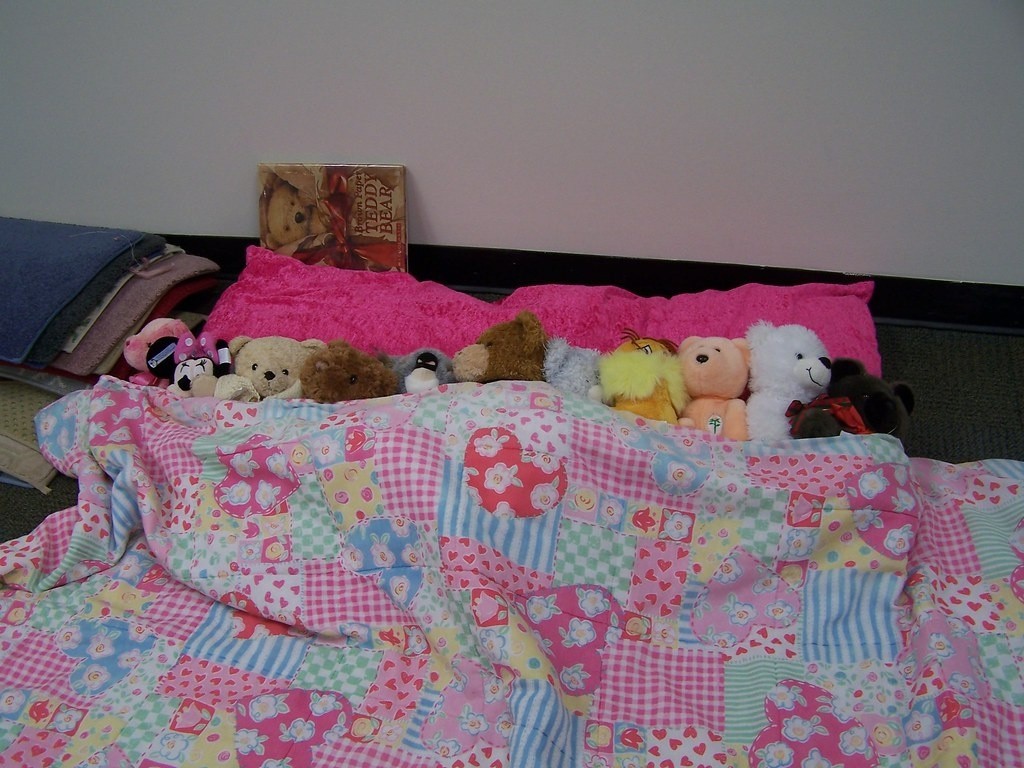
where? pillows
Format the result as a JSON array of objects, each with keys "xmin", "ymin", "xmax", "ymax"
[
  {"xmin": 509, "ymin": 279, "xmax": 883, "ymax": 391},
  {"xmin": 202, "ymin": 245, "xmax": 508, "ymax": 399}
]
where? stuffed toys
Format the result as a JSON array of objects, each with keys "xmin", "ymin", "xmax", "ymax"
[
  {"xmin": 450, "ymin": 309, "xmax": 548, "ymax": 384},
  {"xmin": 743, "ymin": 318, "xmax": 833, "ymax": 442},
  {"xmin": 596, "ymin": 326, "xmax": 694, "ymax": 426},
  {"xmin": 123, "ymin": 317, "xmax": 189, "ymax": 389},
  {"xmin": 374, "ymin": 345, "xmax": 459, "ymax": 395},
  {"xmin": 793, "ymin": 356, "xmax": 916, "ymax": 445},
  {"xmin": 540, "ymin": 332, "xmax": 617, "ymax": 408},
  {"xmin": 213, "ymin": 334, "xmax": 329, "ymax": 404},
  {"xmin": 677, "ymin": 335, "xmax": 753, "ymax": 441},
  {"xmin": 265, "ymin": 173, "xmax": 324, "ymax": 252},
  {"xmin": 145, "ymin": 330, "xmax": 232, "ymax": 398},
  {"xmin": 298, "ymin": 338, "xmax": 400, "ymax": 404}
]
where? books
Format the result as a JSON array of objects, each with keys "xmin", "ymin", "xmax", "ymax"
[{"xmin": 256, "ymin": 161, "xmax": 409, "ymax": 274}]
